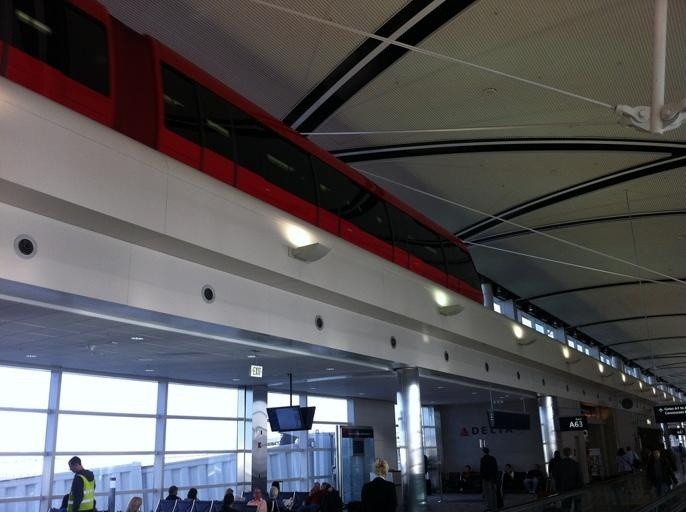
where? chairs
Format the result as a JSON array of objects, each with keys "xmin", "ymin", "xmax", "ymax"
[
  {"xmin": 448, "ymin": 471, "xmax": 544, "ymax": 494},
  {"xmin": 154, "ymin": 492, "xmax": 309, "ymax": 512}
]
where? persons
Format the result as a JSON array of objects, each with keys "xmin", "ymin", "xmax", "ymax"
[
  {"xmin": 66, "ymin": 456, "xmax": 96, "ymax": 512},
  {"xmin": 165, "ymin": 486, "xmax": 181, "ymax": 500},
  {"xmin": 184, "ymin": 488, "xmax": 199, "ymax": 501},
  {"xmin": 361, "ymin": 458, "xmax": 397, "ymax": 512},
  {"xmin": 219, "ymin": 480, "xmax": 343, "ymax": 512},
  {"xmin": 456, "ymin": 443, "xmax": 686, "ymax": 512},
  {"xmin": 126, "ymin": 497, "xmax": 142, "ymax": 512}
]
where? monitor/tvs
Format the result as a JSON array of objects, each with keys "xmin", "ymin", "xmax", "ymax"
[
  {"xmin": 278, "ymin": 406, "xmax": 316, "ymax": 432},
  {"xmin": 266, "ymin": 405, "xmax": 305, "ymax": 432}
]
